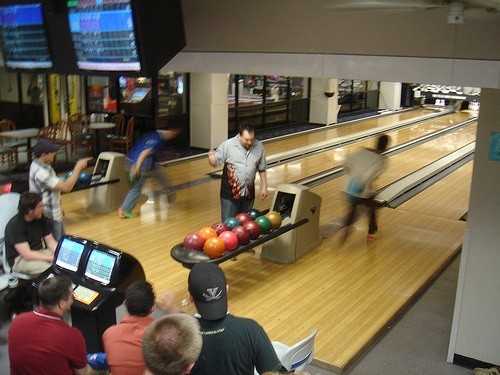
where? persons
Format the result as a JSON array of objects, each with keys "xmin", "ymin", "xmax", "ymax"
[
  {"xmin": 121, "ymin": 124, "xmax": 180, "ymax": 217},
  {"xmin": 8, "ymin": 277, "xmax": 89, "ymax": 375},
  {"xmin": 102, "ymin": 281, "xmax": 174, "ymax": 375},
  {"xmin": 188, "ymin": 262, "xmax": 282, "ymax": 375},
  {"xmin": 141, "ymin": 313, "xmax": 202, "ymax": 375},
  {"xmin": 29, "ymin": 140, "xmax": 93, "ymax": 241},
  {"xmin": 338, "ymin": 134, "xmax": 389, "ymax": 240},
  {"xmin": 5, "ymin": 193, "xmax": 58, "ymax": 278},
  {"xmin": 209, "ymin": 127, "xmax": 268, "ymax": 260}
]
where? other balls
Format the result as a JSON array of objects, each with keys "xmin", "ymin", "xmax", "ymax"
[
  {"xmin": 255, "ymin": 216, "xmax": 272, "ymax": 234},
  {"xmin": 203, "ymin": 238, "xmax": 225, "ymax": 259},
  {"xmin": 236, "ymin": 213, "xmax": 252, "ymax": 226},
  {"xmin": 232, "ymin": 225, "xmax": 250, "ymax": 245},
  {"xmin": 2, "ymin": 183, "xmax": 12, "ymax": 193},
  {"xmin": 211, "ymin": 223, "xmax": 229, "ymax": 237},
  {"xmin": 263, "ymin": 211, "xmax": 282, "ymax": 229},
  {"xmin": 224, "ymin": 217, "xmax": 240, "ymax": 231},
  {"xmin": 198, "ymin": 227, "xmax": 217, "ymax": 241},
  {"xmin": 243, "ymin": 221, "xmax": 261, "ymax": 240},
  {"xmin": 218, "ymin": 231, "xmax": 238, "ymax": 251},
  {"xmin": 247, "ymin": 209, "xmax": 261, "ymax": 220},
  {"xmin": 60, "ymin": 170, "xmax": 91, "ymax": 191},
  {"xmin": 184, "ymin": 232, "xmax": 205, "ymax": 250}
]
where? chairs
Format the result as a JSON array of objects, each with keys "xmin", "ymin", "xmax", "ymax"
[{"xmin": 0, "ymin": 113, "xmax": 134, "ymax": 172}]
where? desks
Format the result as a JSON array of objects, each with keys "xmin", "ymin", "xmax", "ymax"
[
  {"xmin": 0, "ymin": 128, "xmax": 40, "ymax": 171},
  {"xmin": 90, "ymin": 123, "xmax": 117, "ymax": 159}
]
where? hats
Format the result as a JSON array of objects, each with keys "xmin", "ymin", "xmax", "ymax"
[
  {"xmin": 33, "ymin": 139, "xmax": 61, "ymax": 155},
  {"xmin": 188, "ymin": 262, "xmax": 228, "ymax": 321}
]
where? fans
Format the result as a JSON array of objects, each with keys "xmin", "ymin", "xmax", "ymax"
[{"xmin": 329, "ymin": 0, "xmax": 500, "ymax": 25}]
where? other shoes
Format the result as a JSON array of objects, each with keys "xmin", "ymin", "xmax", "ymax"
[
  {"xmin": 118, "ymin": 209, "xmax": 135, "ymax": 218},
  {"xmin": 367, "ymin": 231, "xmax": 381, "ymax": 241}
]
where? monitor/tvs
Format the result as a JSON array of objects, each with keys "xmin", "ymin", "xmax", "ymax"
[
  {"xmin": 83, "ymin": 246, "xmax": 117, "ymax": 285},
  {"xmin": 72, "ymin": 284, "xmax": 99, "ymax": 305},
  {"xmin": 0, "ymin": 0, "xmax": 69, "ymax": 74},
  {"xmin": 66, "ymin": 0, "xmax": 186, "ymax": 76},
  {"xmin": 55, "ymin": 236, "xmax": 85, "ymax": 272}
]
